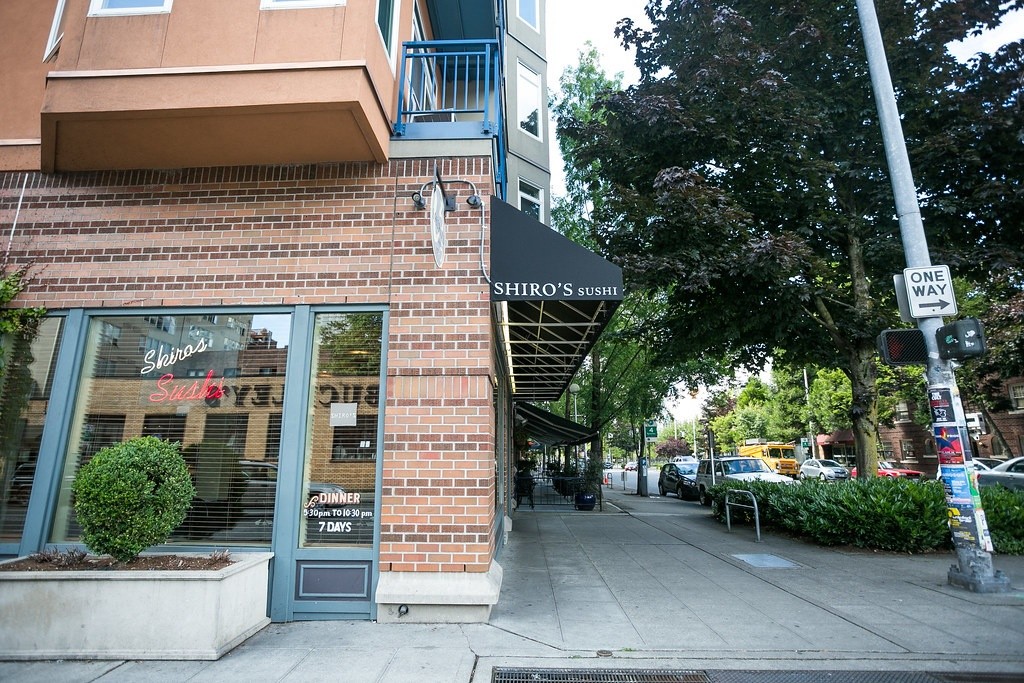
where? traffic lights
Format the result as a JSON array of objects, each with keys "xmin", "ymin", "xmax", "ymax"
[{"xmin": 876, "ymin": 329, "xmax": 929, "ymax": 365}]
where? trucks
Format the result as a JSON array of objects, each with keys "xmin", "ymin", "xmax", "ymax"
[{"xmin": 738, "ymin": 438, "xmax": 800, "ymax": 480}]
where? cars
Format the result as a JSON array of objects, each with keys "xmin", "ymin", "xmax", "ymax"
[
  {"xmin": 851, "ymin": 459, "xmax": 927, "ymax": 484},
  {"xmin": 799, "ymin": 459, "xmax": 851, "ymax": 482},
  {"xmin": 975, "ymin": 456, "xmax": 1024, "ymax": 497},
  {"xmin": 658, "ymin": 462, "xmax": 700, "ymax": 500},
  {"xmin": 603, "ymin": 462, "xmax": 613, "ymax": 469},
  {"xmin": 936, "ymin": 457, "xmax": 1011, "ymax": 482},
  {"xmin": 625, "ymin": 462, "xmax": 638, "ymax": 471},
  {"xmin": 673, "ymin": 456, "xmax": 695, "ymax": 462},
  {"xmin": 234, "ymin": 459, "xmax": 352, "ymax": 522}
]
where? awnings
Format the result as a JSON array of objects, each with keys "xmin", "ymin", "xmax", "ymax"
[
  {"xmin": 513, "ymin": 402, "xmax": 596, "ymax": 451},
  {"xmin": 489, "ymin": 194, "xmax": 622, "ymax": 402}
]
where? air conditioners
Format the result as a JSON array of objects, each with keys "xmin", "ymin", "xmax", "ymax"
[{"xmin": 410, "ymin": 108, "xmax": 457, "ymax": 123}]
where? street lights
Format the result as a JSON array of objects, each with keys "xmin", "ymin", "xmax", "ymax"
[{"xmin": 569, "ymin": 383, "xmax": 580, "ymax": 456}]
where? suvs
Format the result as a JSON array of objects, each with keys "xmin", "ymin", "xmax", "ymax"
[{"xmin": 695, "ymin": 456, "xmax": 802, "ymax": 507}]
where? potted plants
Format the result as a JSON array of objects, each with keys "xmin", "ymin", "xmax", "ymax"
[
  {"xmin": 576, "ymin": 450, "xmax": 605, "ymax": 511},
  {"xmin": 557, "ymin": 462, "xmax": 576, "ymax": 496},
  {"xmin": 514, "ymin": 459, "xmax": 537, "ymax": 510}
]
